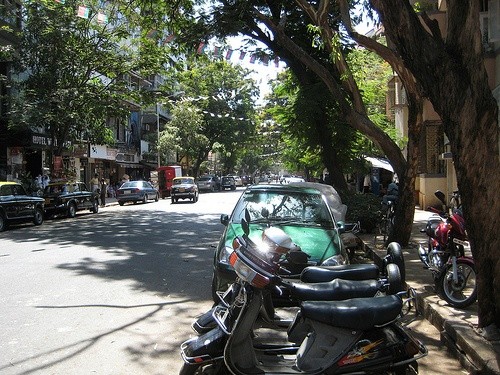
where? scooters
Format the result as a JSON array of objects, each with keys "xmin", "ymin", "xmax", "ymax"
[
  {"xmin": 337, "ymin": 221, "xmax": 361, "ymax": 259},
  {"xmin": 178, "ymin": 205, "xmax": 430, "ymax": 375}
]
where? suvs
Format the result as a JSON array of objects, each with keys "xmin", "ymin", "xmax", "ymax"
[{"xmin": 170, "ymin": 176, "xmax": 199, "ymax": 203}]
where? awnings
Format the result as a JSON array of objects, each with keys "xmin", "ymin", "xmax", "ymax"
[{"xmin": 140, "ymin": 161, "xmax": 160, "ymax": 168}]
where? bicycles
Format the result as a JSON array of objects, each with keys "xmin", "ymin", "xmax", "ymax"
[{"xmin": 449, "ymin": 190, "xmax": 462, "ymax": 209}]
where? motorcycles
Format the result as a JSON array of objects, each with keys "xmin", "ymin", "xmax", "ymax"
[{"xmin": 418, "ymin": 189, "xmax": 479, "ymax": 308}]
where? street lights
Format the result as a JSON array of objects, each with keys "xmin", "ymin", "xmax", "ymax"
[{"xmin": 157, "ymin": 89, "xmax": 187, "ymax": 168}]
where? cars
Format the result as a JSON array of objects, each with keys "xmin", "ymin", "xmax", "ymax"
[
  {"xmin": 41, "ymin": 181, "xmax": 99, "ymax": 219},
  {"xmin": 210, "ymin": 183, "xmax": 351, "ymax": 304},
  {"xmin": 220, "ymin": 176, "xmax": 237, "ymax": 190},
  {"xmin": 0, "ymin": 181, "xmax": 46, "ymax": 233},
  {"xmin": 196, "ymin": 175, "xmax": 222, "ymax": 193},
  {"xmin": 232, "ymin": 173, "xmax": 276, "ymax": 187},
  {"xmin": 279, "ymin": 174, "xmax": 305, "ymax": 184},
  {"xmin": 115, "ymin": 180, "xmax": 160, "ymax": 206}
]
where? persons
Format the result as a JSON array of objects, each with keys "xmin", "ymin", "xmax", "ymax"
[
  {"xmin": 100, "ymin": 178, "xmax": 107, "ymax": 207},
  {"xmin": 383, "ymin": 180, "xmax": 398, "ymax": 205},
  {"xmin": 89, "ymin": 174, "xmax": 99, "ymax": 192}
]
